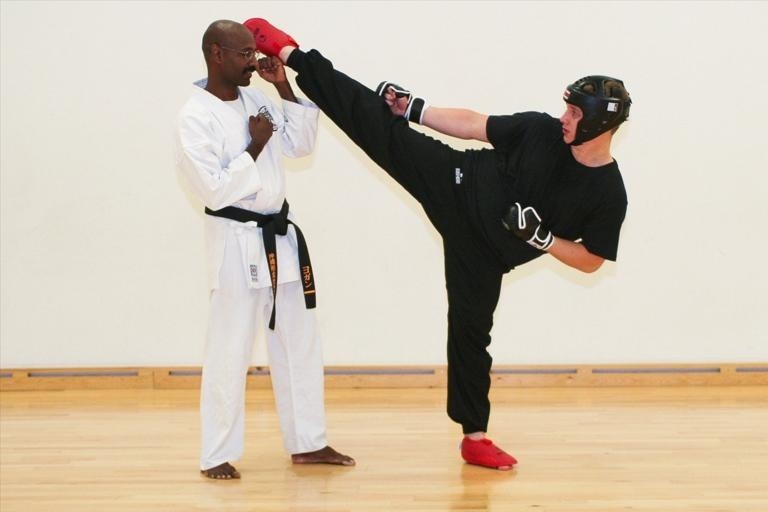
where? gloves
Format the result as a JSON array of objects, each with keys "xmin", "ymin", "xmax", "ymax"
[{"xmin": 500, "ymin": 202, "xmax": 555, "ymax": 251}]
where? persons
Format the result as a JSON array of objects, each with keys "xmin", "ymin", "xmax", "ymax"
[
  {"xmin": 179, "ymin": 19, "xmax": 357, "ymax": 480},
  {"xmin": 241, "ymin": 17, "xmax": 633, "ymax": 471}
]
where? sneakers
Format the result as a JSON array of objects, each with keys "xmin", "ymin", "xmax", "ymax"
[
  {"xmin": 242, "ymin": 18, "xmax": 300, "ymax": 66},
  {"xmin": 459, "ymin": 435, "xmax": 518, "ymax": 471}
]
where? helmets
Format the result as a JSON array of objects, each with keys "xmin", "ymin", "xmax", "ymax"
[{"xmin": 562, "ymin": 74, "xmax": 632, "ymax": 145}]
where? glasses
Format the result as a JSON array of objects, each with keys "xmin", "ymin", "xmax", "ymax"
[{"xmin": 221, "ymin": 46, "xmax": 260, "ymax": 62}]
